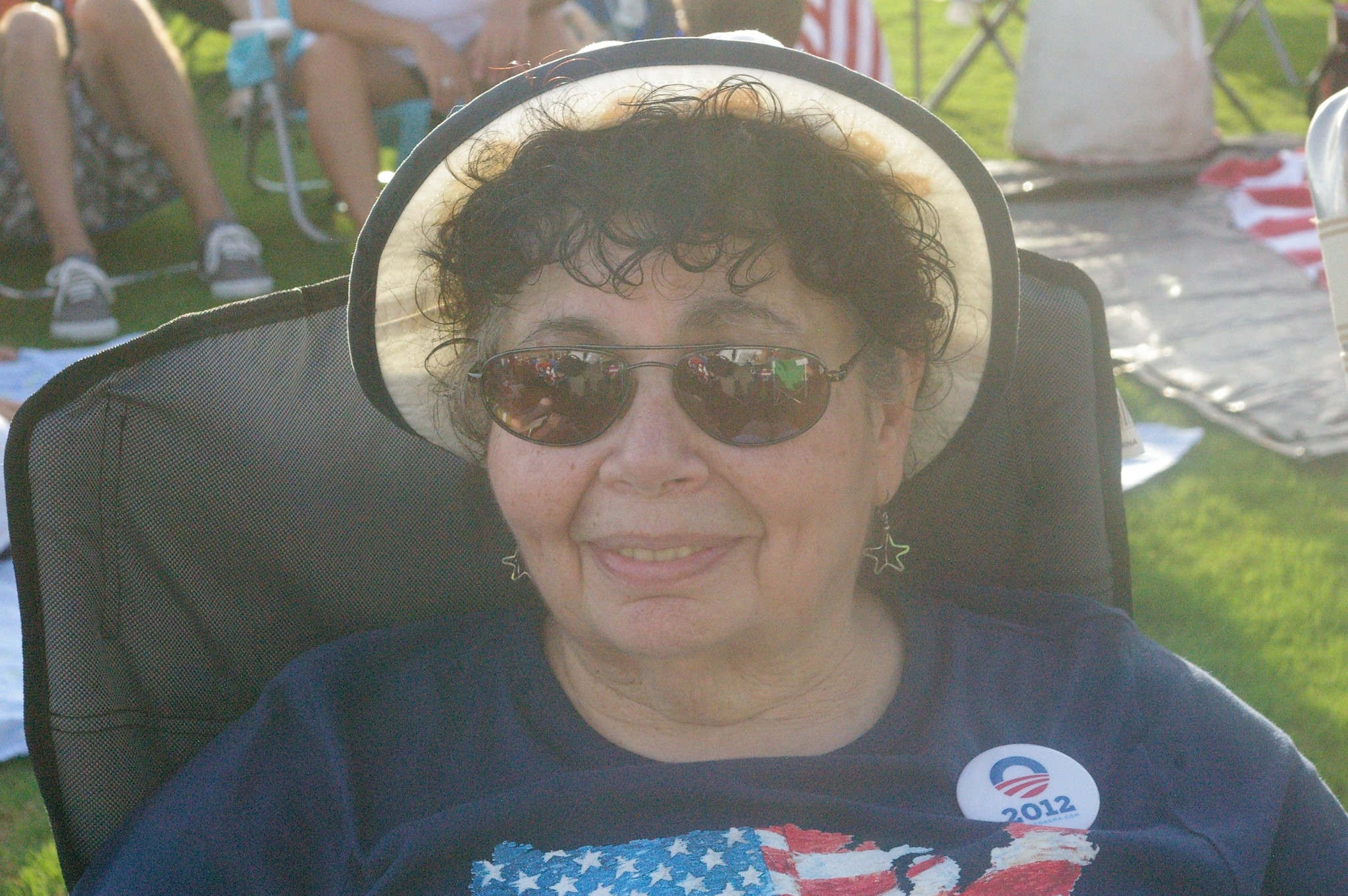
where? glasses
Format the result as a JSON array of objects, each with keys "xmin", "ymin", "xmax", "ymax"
[{"xmin": 477, "ymin": 307, "xmax": 879, "ymax": 449}]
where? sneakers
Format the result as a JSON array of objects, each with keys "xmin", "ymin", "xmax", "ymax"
[
  {"xmin": 193, "ymin": 222, "xmax": 279, "ymax": 296},
  {"xmin": 49, "ymin": 252, "xmax": 123, "ymax": 340}
]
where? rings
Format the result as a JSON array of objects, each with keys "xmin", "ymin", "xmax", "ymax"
[
  {"xmin": 440, "ymin": 77, "xmax": 451, "ymax": 87},
  {"xmin": 428, "ymin": 75, "xmax": 438, "ymax": 84}
]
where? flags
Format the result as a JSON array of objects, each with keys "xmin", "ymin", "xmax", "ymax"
[
  {"xmin": 1195, "ymin": 143, "xmax": 1328, "ymax": 292},
  {"xmin": 789, "ymin": 0, "xmax": 893, "ymax": 91}
]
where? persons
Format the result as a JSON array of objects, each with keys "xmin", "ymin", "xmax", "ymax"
[
  {"xmin": 1304, "ymin": 0, "xmax": 1348, "ymax": 117},
  {"xmin": 0, "ymin": 0, "xmax": 277, "ymax": 342},
  {"xmin": 71, "ymin": 25, "xmax": 1348, "ymax": 896},
  {"xmin": 219, "ymin": 0, "xmax": 575, "ymax": 238}
]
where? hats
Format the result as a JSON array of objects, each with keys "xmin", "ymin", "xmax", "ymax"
[{"xmin": 350, "ymin": 29, "xmax": 1015, "ymax": 500}]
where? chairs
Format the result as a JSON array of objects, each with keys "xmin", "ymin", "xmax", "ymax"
[
  {"xmin": 0, "ymin": 243, "xmax": 1136, "ymax": 894},
  {"xmin": 221, "ymin": 0, "xmax": 379, "ymax": 254}
]
